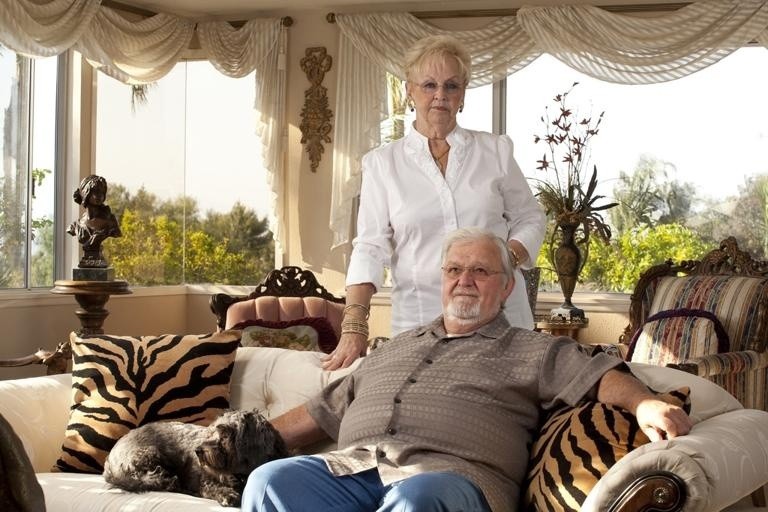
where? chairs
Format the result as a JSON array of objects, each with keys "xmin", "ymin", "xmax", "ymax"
[
  {"xmin": 619, "ymin": 235, "xmax": 766, "ymax": 410},
  {"xmin": 208, "ymin": 267, "xmax": 344, "ymax": 353}
]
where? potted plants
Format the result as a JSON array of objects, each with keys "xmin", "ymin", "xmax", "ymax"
[{"xmin": 524, "ymin": 79, "xmax": 617, "ymax": 323}]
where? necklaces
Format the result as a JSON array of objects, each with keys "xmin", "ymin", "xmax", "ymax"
[{"xmin": 434, "ymin": 147, "xmax": 450, "ymax": 171}]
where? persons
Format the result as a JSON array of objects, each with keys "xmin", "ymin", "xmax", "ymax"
[
  {"xmin": 240, "ymin": 225, "xmax": 691, "ymax": 512},
  {"xmin": 65, "ymin": 175, "xmax": 122, "ymax": 247},
  {"xmin": 320, "ymin": 35, "xmax": 547, "ymax": 371}
]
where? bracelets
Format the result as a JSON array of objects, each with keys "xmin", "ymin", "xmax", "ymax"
[
  {"xmin": 341, "ymin": 303, "xmax": 370, "ymax": 338},
  {"xmin": 508, "ymin": 248, "xmax": 519, "ymax": 267}
]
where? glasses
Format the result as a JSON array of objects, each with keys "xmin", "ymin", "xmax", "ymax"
[
  {"xmin": 408, "ymin": 79, "xmax": 459, "ymax": 94},
  {"xmin": 441, "ymin": 265, "xmax": 507, "ymax": 281}
]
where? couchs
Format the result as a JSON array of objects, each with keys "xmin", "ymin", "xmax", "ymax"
[{"xmin": 0, "ymin": 347, "xmax": 766, "ymax": 512}]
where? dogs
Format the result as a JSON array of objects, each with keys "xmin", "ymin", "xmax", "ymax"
[{"xmin": 100, "ymin": 406, "xmax": 289, "ymax": 509}]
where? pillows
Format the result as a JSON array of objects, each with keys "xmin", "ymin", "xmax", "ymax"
[
  {"xmin": 625, "ymin": 310, "xmax": 729, "ymax": 368},
  {"xmin": 51, "ymin": 320, "xmax": 241, "ymax": 474},
  {"xmin": 523, "ymin": 386, "xmax": 691, "ymax": 512},
  {"xmin": 230, "ymin": 320, "xmax": 339, "ymax": 353}
]
